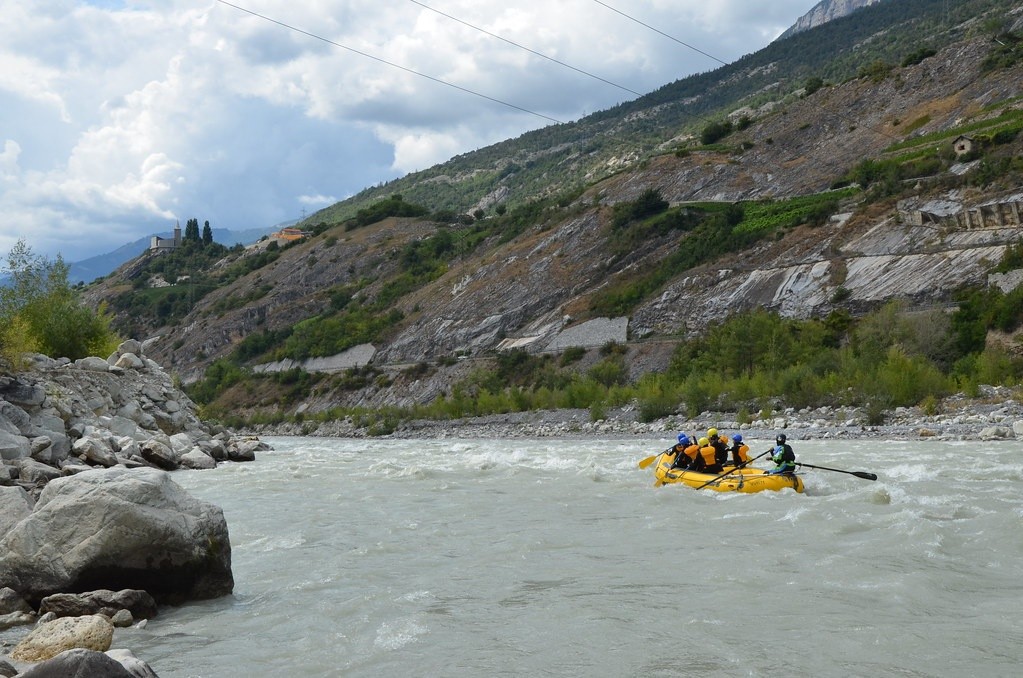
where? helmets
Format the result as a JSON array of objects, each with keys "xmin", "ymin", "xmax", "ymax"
[
  {"xmin": 698, "ymin": 437, "xmax": 709, "ymax": 448},
  {"xmin": 679, "ymin": 437, "xmax": 690, "ymax": 446},
  {"xmin": 776, "ymin": 433, "xmax": 786, "ymax": 444},
  {"xmin": 707, "ymin": 427, "xmax": 717, "ymax": 437},
  {"xmin": 720, "ymin": 436, "xmax": 729, "ymax": 443},
  {"xmin": 732, "ymin": 434, "xmax": 744, "ymax": 441},
  {"xmin": 677, "ymin": 433, "xmax": 686, "ymax": 441}
]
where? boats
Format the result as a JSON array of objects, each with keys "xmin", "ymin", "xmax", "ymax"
[{"xmin": 654, "ymin": 449, "xmax": 804, "ymax": 496}]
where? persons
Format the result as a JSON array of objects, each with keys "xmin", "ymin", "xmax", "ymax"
[
  {"xmin": 762, "ymin": 434, "xmax": 796, "ymax": 475},
  {"xmin": 664, "ymin": 428, "xmax": 748, "ymax": 474}
]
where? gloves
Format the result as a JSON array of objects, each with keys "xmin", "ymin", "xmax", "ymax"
[
  {"xmin": 770, "ymin": 447, "xmax": 774, "ymax": 453},
  {"xmin": 766, "ymin": 457, "xmax": 772, "ymax": 460},
  {"xmin": 725, "ymin": 447, "xmax": 730, "ymax": 451}
]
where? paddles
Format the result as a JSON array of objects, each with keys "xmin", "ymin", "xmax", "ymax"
[
  {"xmin": 653, "ymin": 451, "xmax": 694, "ymax": 489},
  {"xmin": 792, "ymin": 461, "xmax": 878, "ymax": 481},
  {"xmin": 639, "ymin": 435, "xmax": 691, "ymax": 470},
  {"xmin": 695, "ymin": 449, "xmax": 770, "ymax": 490}
]
